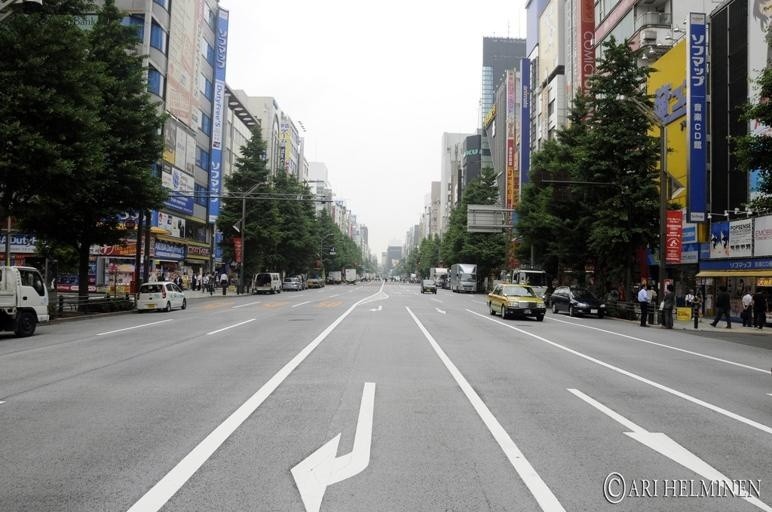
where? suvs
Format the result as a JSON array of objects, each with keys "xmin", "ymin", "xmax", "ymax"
[{"xmin": 421, "ymin": 279, "xmax": 438, "ymax": 294}]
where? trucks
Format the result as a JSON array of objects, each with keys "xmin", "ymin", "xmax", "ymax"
[
  {"xmin": 0, "ymin": 265, "xmax": 51, "ymax": 337},
  {"xmin": 428, "ymin": 267, "xmax": 451, "ymax": 288},
  {"xmin": 451, "ymin": 263, "xmax": 479, "ymax": 293},
  {"xmin": 511, "ymin": 269, "xmax": 549, "ymax": 306}
]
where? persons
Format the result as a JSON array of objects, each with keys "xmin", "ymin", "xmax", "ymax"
[
  {"xmin": 549, "ymin": 272, "xmax": 768, "ymax": 330},
  {"xmin": 147, "ymin": 268, "xmax": 239, "ymax": 297}
]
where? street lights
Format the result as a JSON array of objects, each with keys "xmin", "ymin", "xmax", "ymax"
[
  {"xmin": 320, "ymin": 233, "xmax": 333, "ymax": 265},
  {"xmin": 241, "ymin": 182, "xmax": 272, "ymax": 291},
  {"xmin": 614, "ymin": 94, "xmax": 666, "ymax": 309}
]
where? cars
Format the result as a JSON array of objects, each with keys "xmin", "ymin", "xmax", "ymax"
[
  {"xmin": 486, "ymin": 283, "xmax": 547, "ymax": 322},
  {"xmin": 549, "ymin": 286, "xmax": 608, "ymax": 317},
  {"xmin": 135, "ymin": 282, "xmax": 185, "ymax": 311},
  {"xmin": 253, "ymin": 268, "xmax": 377, "ymax": 294}
]
